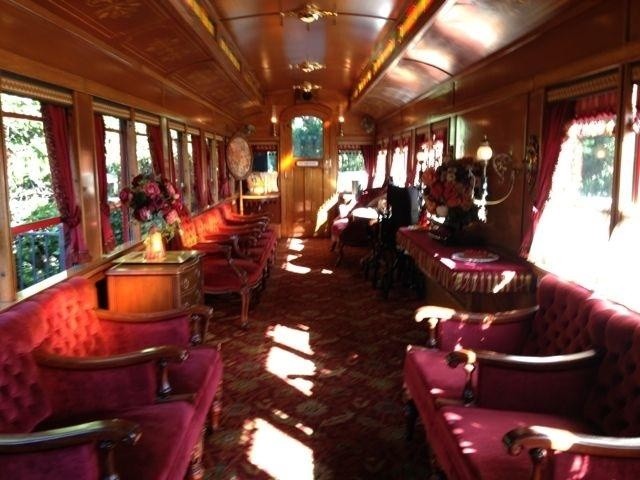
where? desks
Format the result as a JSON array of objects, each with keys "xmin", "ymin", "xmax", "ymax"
[{"xmin": 397, "ymin": 223, "xmax": 533, "ymax": 312}]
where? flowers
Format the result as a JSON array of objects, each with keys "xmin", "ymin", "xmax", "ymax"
[
  {"xmin": 118, "ymin": 160, "xmax": 185, "ymax": 237},
  {"xmin": 417, "ymin": 149, "xmax": 487, "ymax": 217}
]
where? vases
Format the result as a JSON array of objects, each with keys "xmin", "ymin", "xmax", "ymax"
[
  {"xmin": 142, "ymin": 232, "xmax": 167, "ymax": 260},
  {"xmin": 444, "ymin": 204, "xmax": 481, "ymax": 233}
]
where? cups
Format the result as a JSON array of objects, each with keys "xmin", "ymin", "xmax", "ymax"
[{"xmin": 351, "ymin": 181, "xmax": 360, "ymax": 196}]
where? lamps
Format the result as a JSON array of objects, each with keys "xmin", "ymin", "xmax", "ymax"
[
  {"xmin": 287, "ymin": 59, "xmax": 326, "ymax": 74},
  {"xmin": 464, "ymin": 133, "xmax": 539, "ymax": 225},
  {"xmin": 278, "ymin": 1, "xmax": 341, "ymax": 25},
  {"xmin": 293, "ymin": 81, "xmax": 321, "ymax": 100}
]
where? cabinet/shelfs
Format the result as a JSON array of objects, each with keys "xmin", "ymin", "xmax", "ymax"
[{"xmin": 105, "ymin": 249, "xmax": 207, "ymax": 310}]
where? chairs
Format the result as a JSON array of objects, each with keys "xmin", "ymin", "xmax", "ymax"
[
  {"xmin": 160, "ymin": 196, "xmax": 281, "ymax": 330},
  {"xmin": 0, "ymin": 277, "xmax": 223, "ymax": 480},
  {"xmin": 399, "ymin": 271, "xmax": 639, "ymax": 479},
  {"xmin": 331, "ymin": 187, "xmax": 417, "ymax": 280}
]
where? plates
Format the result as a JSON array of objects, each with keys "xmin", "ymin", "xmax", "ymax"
[{"xmin": 452, "ymin": 252, "xmax": 499, "ymax": 263}]
